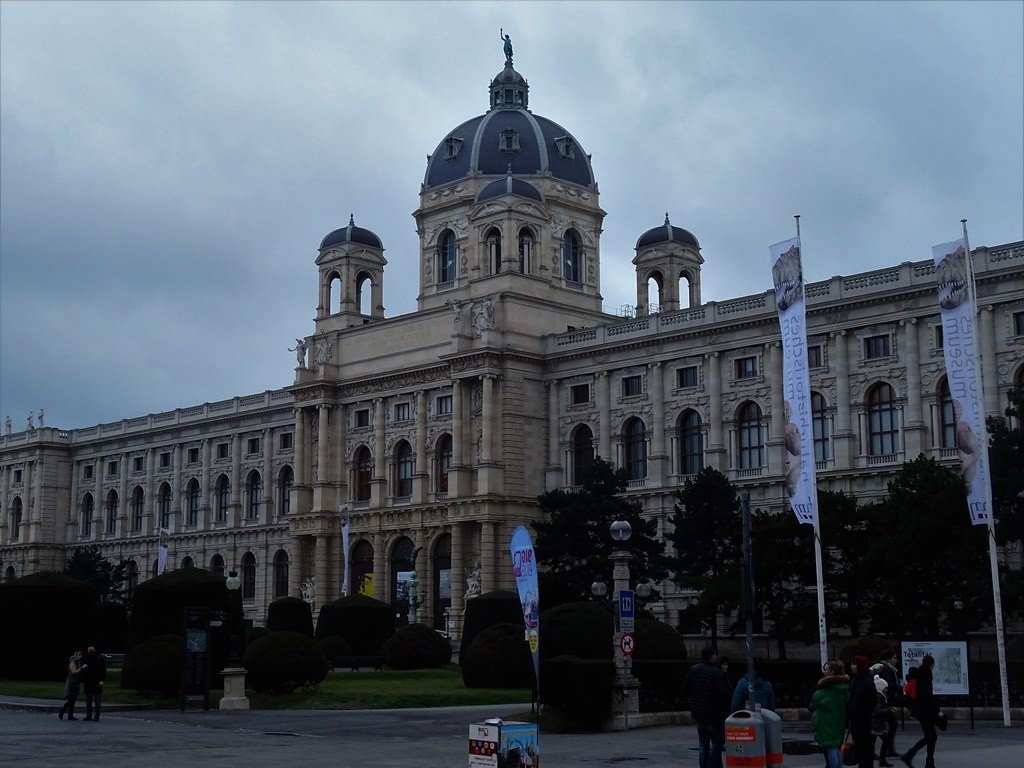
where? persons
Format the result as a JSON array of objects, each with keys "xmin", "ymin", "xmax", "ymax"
[
  {"xmin": 809, "ymin": 649, "xmax": 901, "ymax": 768},
  {"xmin": 27, "ymin": 411, "xmax": 33, "ymax": 424},
  {"xmin": 685, "ymin": 646, "xmax": 731, "ymax": 768},
  {"xmin": 500, "ymin": 28, "xmax": 513, "ymax": 60},
  {"xmin": 465, "ymin": 560, "xmax": 482, "ymax": 592},
  {"xmin": 300, "ymin": 577, "xmax": 314, "ymax": 599},
  {"xmin": 82, "ymin": 645, "xmax": 106, "ymax": 722},
  {"xmin": 288, "ymin": 339, "xmax": 308, "ymax": 367},
  {"xmin": 716, "ymin": 656, "xmax": 733, "ymax": 752},
  {"xmin": 482, "ymin": 297, "xmax": 491, "ymax": 325},
  {"xmin": 38, "ymin": 408, "xmax": 44, "ymax": 427},
  {"xmin": 900, "ymin": 655, "xmax": 944, "ymax": 768},
  {"xmin": 5, "ymin": 416, "xmax": 11, "ymax": 433},
  {"xmin": 733, "ymin": 659, "xmax": 776, "ymax": 713},
  {"xmin": 59, "ymin": 651, "xmax": 88, "ymax": 720}
]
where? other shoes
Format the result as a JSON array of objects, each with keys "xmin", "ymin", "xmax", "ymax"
[
  {"xmin": 879, "ymin": 760, "xmax": 893, "ymax": 767},
  {"xmin": 83, "ymin": 716, "xmax": 92, "ymax": 721},
  {"xmin": 874, "ymin": 753, "xmax": 879, "ymax": 760},
  {"xmin": 888, "ymin": 751, "xmax": 900, "ymax": 757},
  {"xmin": 68, "ymin": 715, "xmax": 78, "ymax": 720},
  {"xmin": 59, "ymin": 710, "xmax": 63, "ymax": 719},
  {"xmin": 91, "ymin": 717, "xmax": 99, "ymax": 721}
]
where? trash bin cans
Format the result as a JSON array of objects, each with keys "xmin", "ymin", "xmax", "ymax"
[
  {"xmin": 761, "ymin": 708, "xmax": 783, "ymax": 768},
  {"xmin": 725, "ymin": 710, "xmax": 766, "ymax": 768}
]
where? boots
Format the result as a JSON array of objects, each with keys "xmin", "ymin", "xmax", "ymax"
[
  {"xmin": 925, "ymin": 758, "xmax": 935, "ymax": 768},
  {"xmin": 900, "ymin": 749, "xmax": 916, "ymax": 768}
]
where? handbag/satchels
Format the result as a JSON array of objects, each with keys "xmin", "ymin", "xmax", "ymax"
[
  {"xmin": 904, "ymin": 678, "xmax": 917, "ymax": 700},
  {"xmin": 841, "ymin": 741, "xmax": 858, "ymax": 766}
]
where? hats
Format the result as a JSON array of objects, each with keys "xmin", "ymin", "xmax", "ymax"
[
  {"xmin": 854, "ymin": 656, "xmax": 869, "ymax": 674},
  {"xmin": 873, "ymin": 674, "xmax": 888, "ymax": 703}
]
